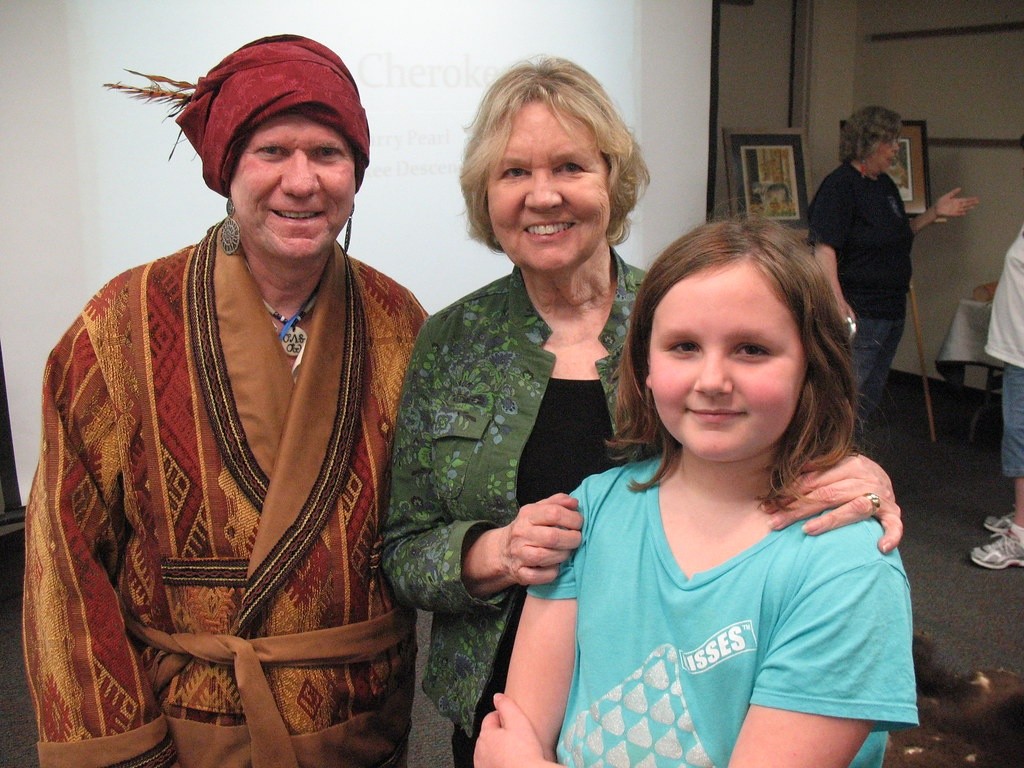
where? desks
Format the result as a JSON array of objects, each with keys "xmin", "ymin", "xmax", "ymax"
[{"xmin": 936, "ymin": 282, "xmax": 1005, "ymax": 440}]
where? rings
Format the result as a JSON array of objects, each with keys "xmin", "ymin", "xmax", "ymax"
[{"xmin": 865, "ymin": 492, "xmax": 880, "ymax": 516}]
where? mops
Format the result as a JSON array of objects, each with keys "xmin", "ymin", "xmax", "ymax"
[{"xmin": 907, "ymin": 278, "xmax": 966, "ymax": 464}]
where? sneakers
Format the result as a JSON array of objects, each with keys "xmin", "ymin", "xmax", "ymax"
[
  {"xmin": 983, "ymin": 504, "xmax": 1017, "ymax": 534},
  {"xmin": 971, "ymin": 528, "xmax": 1024, "ymax": 569}
]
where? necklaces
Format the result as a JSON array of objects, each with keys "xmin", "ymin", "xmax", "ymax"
[{"xmin": 261, "ymin": 295, "xmax": 316, "ymax": 358}]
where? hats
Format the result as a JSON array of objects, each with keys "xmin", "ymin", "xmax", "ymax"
[{"xmin": 102, "ymin": 34, "xmax": 369, "ymax": 198}]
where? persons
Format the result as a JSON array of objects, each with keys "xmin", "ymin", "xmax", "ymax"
[
  {"xmin": 21, "ymin": 33, "xmax": 429, "ymax": 767},
  {"xmin": 473, "ymin": 217, "xmax": 921, "ymax": 767},
  {"xmin": 808, "ymin": 104, "xmax": 1024, "ymax": 569},
  {"xmin": 387, "ymin": 57, "xmax": 904, "ymax": 768}
]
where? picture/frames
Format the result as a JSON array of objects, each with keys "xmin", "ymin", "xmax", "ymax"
[
  {"xmin": 839, "ymin": 119, "xmax": 931, "ymax": 217},
  {"xmin": 722, "ymin": 126, "xmax": 814, "ymax": 241}
]
